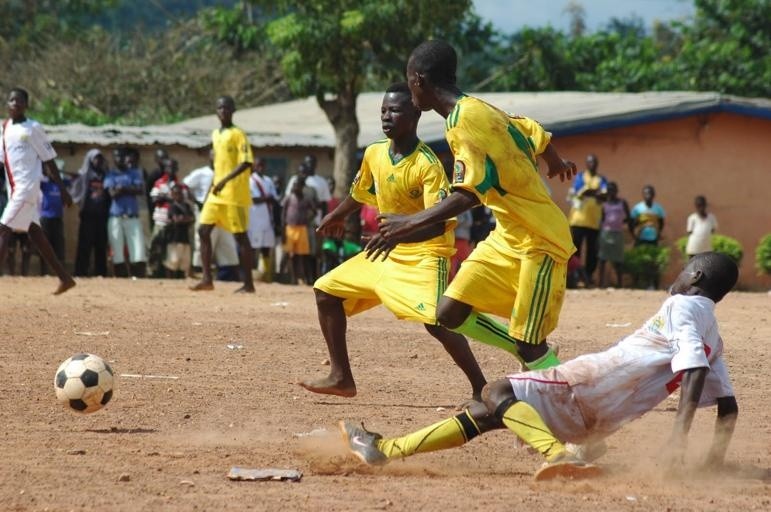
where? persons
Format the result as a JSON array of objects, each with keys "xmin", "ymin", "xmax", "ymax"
[
  {"xmin": 0, "ymin": 87, "xmax": 76, "ymax": 296},
  {"xmin": 182, "ymin": 146, "xmax": 240, "ymax": 281},
  {"xmin": 323, "ymin": 174, "xmax": 346, "ymax": 278},
  {"xmin": 284, "ymin": 154, "xmax": 333, "ymax": 227},
  {"xmin": 149, "ymin": 157, "xmax": 186, "ymax": 239},
  {"xmin": 567, "ymin": 254, "xmax": 594, "ymax": 288},
  {"xmin": 149, "ymin": 184, "xmax": 195, "ymax": 280},
  {"xmin": 685, "ymin": 196, "xmax": 718, "ymax": 260},
  {"xmin": 0, "ymin": 164, "xmax": 32, "ymax": 276},
  {"xmin": 601, "ymin": 181, "xmax": 631, "ymax": 288},
  {"xmin": 70, "ymin": 147, "xmax": 111, "ymax": 277},
  {"xmin": 102, "ymin": 146, "xmax": 148, "ymax": 278},
  {"xmin": 248, "ymin": 157, "xmax": 280, "ymax": 283},
  {"xmin": 565, "ymin": 151, "xmax": 609, "ymax": 285},
  {"xmin": 372, "ymin": 40, "xmax": 578, "ymax": 373},
  {"xmin": 279, "ymin": 176, "xmax": 318, "ymax": 285},
  {"xmin": 39, "ymin": 167, "xmax": 81, "ymax": 276},
  {"xmin": 297, "ymin": 82, "xmax": 488, "ymax": 412},
  {"xmin": 123, "ymin": 147, "xmax": 152, "ymax": 241},
  {"xmin": 271, "ymin": 173, "xmax": 284, "ymax": 237},
  {"xmin": 187, "ymin": 94, "xmax": 257, "ymax": 294},
  {"xmin": 628, "ymin": 185, "xmax": 666, "ymax": 290},
  {"xmin": 296, "ymin": 163, "xmax": 323, "ymax": 285},
  {"xmin": 338, "ymin": 250, "xmax": 740, "ymax": 484},
  {"xmin": 146, "ymin": 147, "xmax": 169, "ymax": 231}
]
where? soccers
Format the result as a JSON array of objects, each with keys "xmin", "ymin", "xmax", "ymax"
[{"xmin": 52, "ymin": 353, "xmax": 116, "ymax": 415}]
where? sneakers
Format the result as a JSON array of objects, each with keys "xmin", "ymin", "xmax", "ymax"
[
  {"xmin": 338, "ymin": 420, "xmax": 389, "ymax": 469},
  {"xmin": 533, "ymin": 452, "xmax": 597, "ymax": 483}
]
